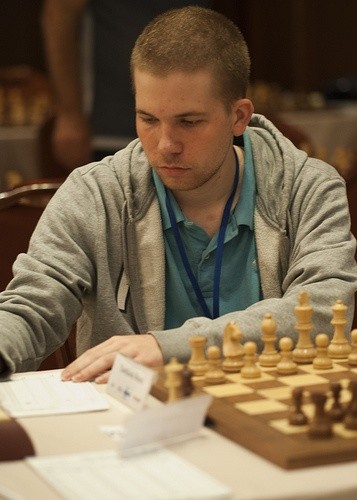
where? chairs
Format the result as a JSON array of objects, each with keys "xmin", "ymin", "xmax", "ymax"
[{"xmin": 0, "ymin": 177, "xmax": 63, "ymax": 298}]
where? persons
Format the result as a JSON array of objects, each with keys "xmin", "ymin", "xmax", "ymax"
[{"xmin": 0, "ymin": 7, "xmax": 357, "ymax": 386}]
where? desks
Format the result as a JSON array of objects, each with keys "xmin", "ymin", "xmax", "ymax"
[{"xmin": 0, "ymin": 368, "xmax": 357, "ymax": 499}]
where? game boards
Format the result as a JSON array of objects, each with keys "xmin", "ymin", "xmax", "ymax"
[{"xmin": 155, "ymin": 324, "xmax": 357, "ymax": 470}]
